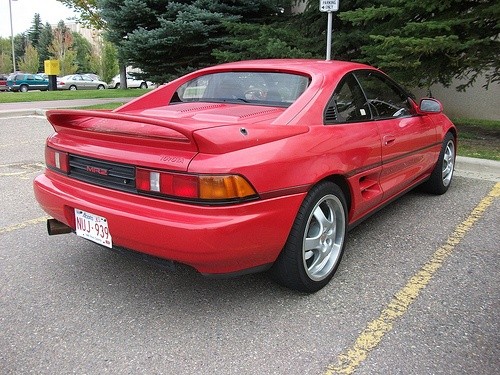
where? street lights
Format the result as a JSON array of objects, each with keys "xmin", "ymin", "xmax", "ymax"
[{"xmin": 9, "ymin": 0, "xmax": 18, "ymax": 71}]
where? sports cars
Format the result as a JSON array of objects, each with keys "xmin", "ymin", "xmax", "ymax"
[{"xmin": 31, "ymin": 59, "xmax": 459, "ymax": 292}]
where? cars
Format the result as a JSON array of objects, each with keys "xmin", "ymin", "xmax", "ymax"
[
  {"xmin": 56, "ymin": 74, "xmax": 108, "ymax": 91},
  {"xmin": 112, "ymin": 71, "xmax": 156, "ymax": 90},
  {"xmin": 0, "ymin": 74, "xmax": 50, "ymax": 92}
]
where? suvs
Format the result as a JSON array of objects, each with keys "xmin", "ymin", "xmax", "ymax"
[{"xmin": 6, "ymin": 72, "xmax": 50, "ymax": 93}]
